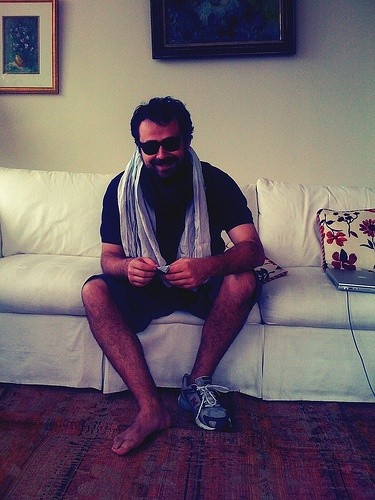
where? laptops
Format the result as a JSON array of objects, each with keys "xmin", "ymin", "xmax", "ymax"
[{"xmin": 325, "ymin": 268, "xmax": 375, "ymax": 293}]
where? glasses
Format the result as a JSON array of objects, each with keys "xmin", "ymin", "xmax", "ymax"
[{"xmin": 136, "ymin": 136, "xmax": 185, "ymax": 156}]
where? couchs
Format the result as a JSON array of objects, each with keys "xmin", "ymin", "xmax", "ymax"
[{"xmin": 0, "ymin": 169, "xmax": 375, "ymax": 406}]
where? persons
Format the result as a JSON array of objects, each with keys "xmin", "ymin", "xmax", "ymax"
[{"xmin": 80, "ymin": 96, "xmax": 266, "ymax": 456}]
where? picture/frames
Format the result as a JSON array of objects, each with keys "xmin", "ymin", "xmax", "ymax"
[
  {"xmin": 0, "ymin": 0, "xmax": 60, "ymax": 94},
  {"xmin": 146, "ymin": 0, "xmax": 297, "ymax": 60}
]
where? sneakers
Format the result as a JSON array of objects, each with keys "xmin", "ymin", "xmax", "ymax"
[{"xmin": 174, "ymin": 373, "xmax": 235, "ymax": 434}]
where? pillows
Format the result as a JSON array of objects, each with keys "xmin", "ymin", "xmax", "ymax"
[
  {"xmin": 224, "ymin": 238, "xmax": 289, "ymax": 283},
  {"xmin": 316, "ymin": 205, "xmax": 375, "ymax": 273}
]
